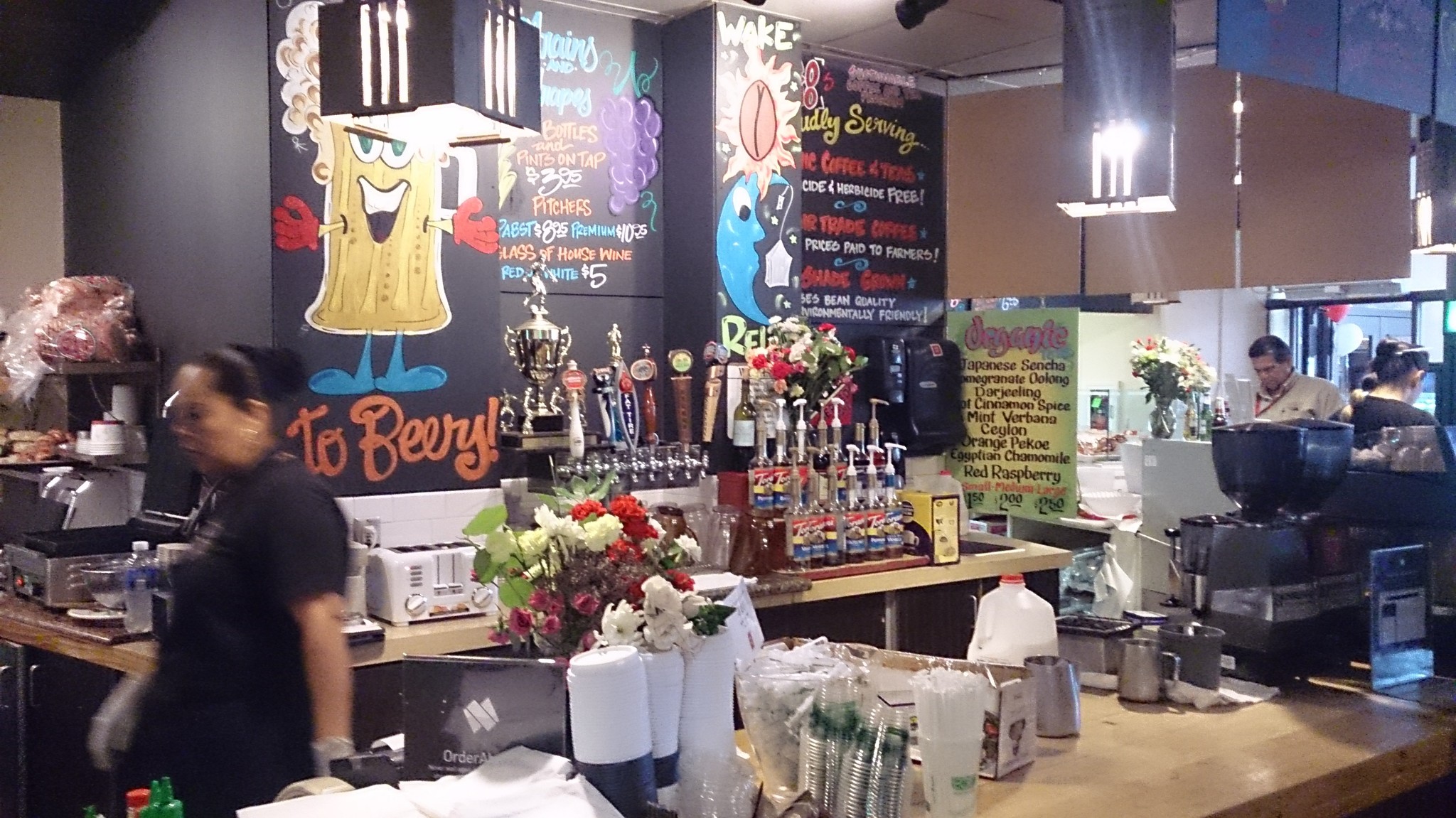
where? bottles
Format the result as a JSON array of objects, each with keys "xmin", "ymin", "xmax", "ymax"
[
  {"xmin": 849, "ymin": 422, "xmax": 872, "ymax": 501},
  {"xmin": 966, "ymin": 572, "xmax": 1058, "ymax": 666},
  {"xmin": 1184, "ymin": 385, "xmax": 1231, "ymax": 442},
  {"xmin": 124, "ymin": 540, "xmax": 155, "ymax": 633},
  {"xmin": 732, "ymin": 379, "xmax": 757, "ymax": 471},
  {"xmin": 933, "ymin": 470, "xmax": 970, "ymax": 537},
  {"xmin": 126, "ymin": 777, "xmax": 184, "ymax": 818},
  {"xmin": 889, "ymin": 431, "xmax": 906, "ymax": 490}
]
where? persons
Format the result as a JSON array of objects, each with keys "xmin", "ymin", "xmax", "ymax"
[
  {"xmin": 1247, "ymin": 333, "xmax": 1341, "ymax": 426},
  {"xmin": 1340, "ymin": 388, "xmax": 1367, "ymax": 422},
  {"xmin": 1350, "ymin": 335, "xmax": 1456, "ymax": 470},
  {"xmin": 1360, "ymin": 372, "xmax": 1377, "ymax": 392},
  {"xmin": 89, "ymin": 341, "xmax": 353, "ymax": 815}
]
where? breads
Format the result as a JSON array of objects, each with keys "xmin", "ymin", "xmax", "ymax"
[
  {"xmin": 32, "ymin": 274, "xmax": 143, "ymax": 363},
  {"xmin": 0, "ymin": 428, "xmax": 77, "ymax": 463}
]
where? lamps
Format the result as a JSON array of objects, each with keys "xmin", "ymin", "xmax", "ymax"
[{"xmin": 314, "ymin": 0, "xmax": 543, "ymax": 148}]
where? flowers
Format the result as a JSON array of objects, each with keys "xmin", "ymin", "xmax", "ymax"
[
  {"xmin": 743, "ymin": 315, "xmax": 871, "ymax": 410},
  {"xmin": 458, "ymin": 469, "xmax": 743, "ymax": 652}
]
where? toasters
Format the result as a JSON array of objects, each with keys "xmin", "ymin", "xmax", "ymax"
[{"xmin": 366, "ymin": 540, "xmax": 500, "ymax": 627}]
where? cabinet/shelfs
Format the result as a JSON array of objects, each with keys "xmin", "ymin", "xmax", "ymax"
[{"xmin": 0, "ymin": 347, "xmax": 160, "ymax": 468}]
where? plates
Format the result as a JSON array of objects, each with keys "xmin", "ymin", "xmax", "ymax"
[
  {"xmin": 157, "ymin": 542, "xmax": 196, "ymax": 567},
  {"xmin": 66, "ymin": 608, "xmax": 125, "ymax": 622}
]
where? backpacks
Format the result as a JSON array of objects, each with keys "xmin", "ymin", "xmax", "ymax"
[{"xmin": 905, "ymin": 337, "xmax": 967, "ymax": 456}]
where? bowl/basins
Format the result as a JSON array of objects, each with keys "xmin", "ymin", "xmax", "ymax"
[{"xmin": 80, "ymin": 558, "xmax": 126, "ymax": 612}]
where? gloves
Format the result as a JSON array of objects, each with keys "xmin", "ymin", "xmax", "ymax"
[
  {"xmin": 308, "ymin": 735, "xmax": 357, "ymax": 776},
  {"xmin": 87, "ymin": 672, "xmax": 144, "ymax": 774}
]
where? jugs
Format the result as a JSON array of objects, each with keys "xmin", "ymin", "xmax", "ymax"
[
  {"xmin": 1024, "ymin": 655, "xmax": 1082, "ymax": 737},
  {"xmin": 1116, "ymin": 636, "xmax": 1181, "ymax": 703}
]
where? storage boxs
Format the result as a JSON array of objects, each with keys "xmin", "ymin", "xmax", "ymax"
[{"xmin": 720, "ymin": 626, "xmax": 1043, "ymax": 780}]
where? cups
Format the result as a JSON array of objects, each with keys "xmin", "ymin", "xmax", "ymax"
[
  {"xmin": 1160, "ymin": 623, "xmax": 1225, "ymax": 693},
  {"xmin": 734, "ymin": 649, "xmax": 983, "ymax": 818},
  {"xmin": 565, "ymin": 625, "xmax": 756, "ymax": 818},
  {"xmin": 645, "ymin": 501, "xmax": 776, "ymax": 578},
  {"xmin": 499, "ymin": 477, "xmax": 529, "ymax": 524},
  {"xmin": 75, "ymin": 382, "xmax": 148, "ymax": 455}
]
